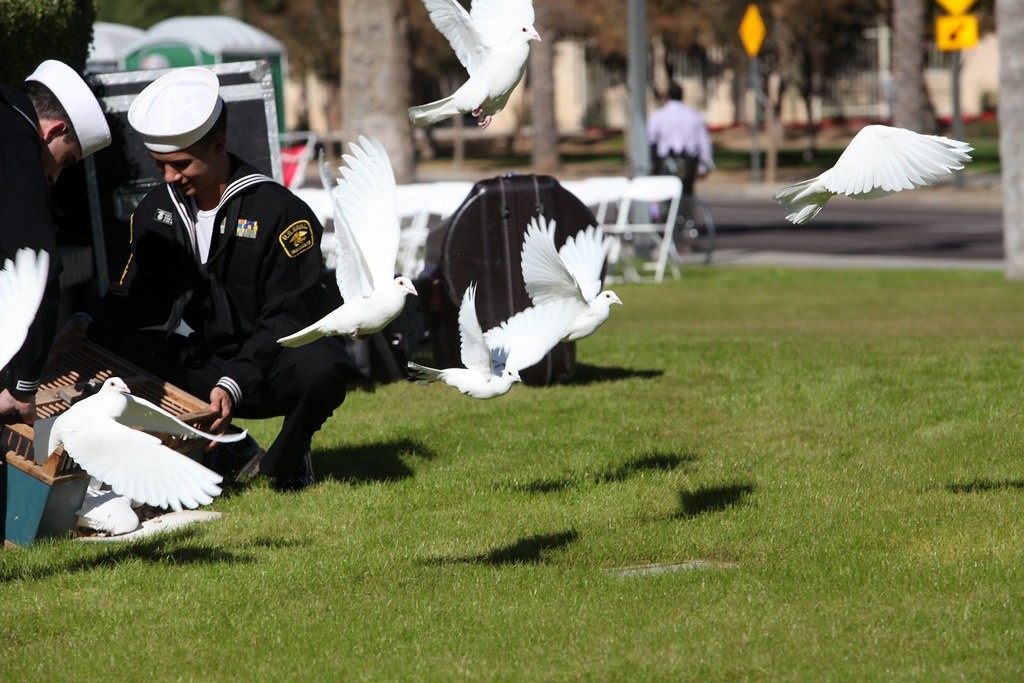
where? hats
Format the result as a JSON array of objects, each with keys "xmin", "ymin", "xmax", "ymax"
[
  {"xmin": 24, "ymin": 58, "xmax": 112, "ymax": 160},
  {"xmin": 127, "ymin": 67, "xmax": 224, "ymax": 154}
]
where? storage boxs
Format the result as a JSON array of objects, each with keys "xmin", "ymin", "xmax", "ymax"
[
  {"xmin": 115, "ymin": 13, "xmax": 288, "ymax": 148},
  {"xmin": 57, "ymin": 54, "xmax": 284, "ymax": 307}
]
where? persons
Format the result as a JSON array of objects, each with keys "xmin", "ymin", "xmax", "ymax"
[
  {"xmin": 0, "ymin": 59, "xmax": 108, "ymax": 427},
  {"xmin": 53, "ymin": 67, "xmax": 343, "ymax": 497},
  {"xmin": 650, "ymin": 83, "xmax": 718, "ymax": 217}
]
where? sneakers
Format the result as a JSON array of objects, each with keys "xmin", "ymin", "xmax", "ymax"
[
  {"xmin": 276, "ymin": 451, "xmax": 315, "ymax": 493},
  {"xmin": 200, "ymin": 427, "xmax": 266, "ymax": 489}
]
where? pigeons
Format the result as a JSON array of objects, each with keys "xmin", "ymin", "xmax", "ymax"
[
  {"xmin": 276, "ymin": 132, "xmax": 417, "ymax": 348},
  {"xmin": 0, "ymin": 248, "xmax": 49, "ymax": 376},
  {"xmin": 771, "ymin": 123, "xmax": 977, "ymax": 225},
  {"xmin": 406, "ymin": 0, "xmax": 542, "ymax": 130},
  {"xmin": 32, "ymin": 377, "xmax": 249, "ymax": 537},
  {"xmin": 408, "ymin": 211, "xmax": 625, "ymax": 400}
]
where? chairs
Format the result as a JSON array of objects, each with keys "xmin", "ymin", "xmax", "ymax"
[
  {"xmin": 394, "ymin": 184, "xmax": 431, "ymax": 278},
  {"xmin": 601, "ymin": 175, "xmax": 683, "ymax": 284},
  {"xmin": 572, "ymin": 175, "xmax": 639, "ymax": 284},
  {"xmin": 404, "ymin": 180, "xmax": 474, "ymax": 277}
]
